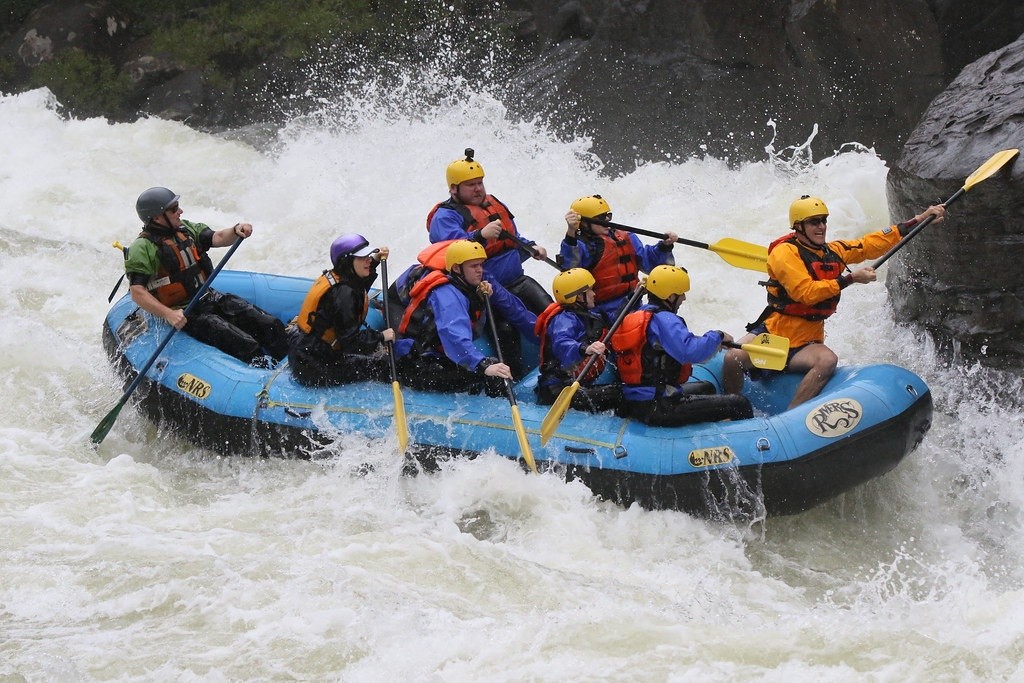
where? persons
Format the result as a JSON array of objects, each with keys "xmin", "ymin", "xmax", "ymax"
[
  {"xmin": 722, "ymin": 195, "xmax": 947, "ymax": 411},
  {"xmin": 124, "ymin": 187, "xmax": 289, "ymax": 371},
  {"xmin": 387, "ymin": 236, "xmax": 545, "ymax": 401},
  {"xmin": 286, "ymin": 232, "xmax": 400, "ymax": 389},
  {"xmin": 619, "ymin": 264, "xmax": 754, "ymax": 428},
  {"xmin": 535, "ymin": 267, "xmax": 648, "ymax": 416},
  {"xmin": 426, "ymin": 159, "xmax": 555, "ymax": 385},
  {"xmin": 559, "ymin": 194, "xmax": 678, "ymax": 324}
]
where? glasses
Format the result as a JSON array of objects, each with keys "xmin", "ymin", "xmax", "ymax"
[
  {"xmin": 798, "ymin": 216, "xmax": 827, "ymax": 225},
  {"xmin": 166, "ymin": 204, "xmax": 178, "ymax": 213},
  {"xmin": 593, "ymin": 212, "xmax": 612, "ymax": 220}
]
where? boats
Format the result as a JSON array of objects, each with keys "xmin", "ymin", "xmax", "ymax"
[{"xmin": 102, "ymin": 270, "xmax": 933, "ymax": 526}]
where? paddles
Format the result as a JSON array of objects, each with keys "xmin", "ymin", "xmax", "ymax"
[
  {"xmin": 83, "ymin": 228, "xmax": 253, "ymax": 451},
  {"xmin": 378, "ymin": 247, "xmax": 411, "ymax": 451},
  {"xmin": 570, "ymin": 209, "xmax": 770, "ymax": 273},
  {"xmin": 870, "ymin": 147, "xmax": 1022, "ymax": 271},
  {"xmin": 478, "ymin": 282, "xmax": 541, "ymax": 478},
  {"xmin": 720, "ymin": 332, "xmax": 791, "ymax": 371},
  {"xmin": 500, "ymin": 227, "xmax": 563, "ymax": 271},
  {"xmin": 539, "ymin": 276, "xmax": 647, "ymax": 449}
]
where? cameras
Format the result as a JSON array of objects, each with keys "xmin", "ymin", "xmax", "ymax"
[{"xmin": 465, "ymin": 148, "xmax": 474, "ymax": 159}]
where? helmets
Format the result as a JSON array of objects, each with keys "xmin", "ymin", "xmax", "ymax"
[
  {"xmin": 446, "ymin": 148, "xmax": 485, "ymax": 189},
  {"xmin": 645, "ymin": 264, "xmax": 690, "ymax": 299},
  {"xmin": 789, "ymin": 195, "xmax": 829, "ymax": 228},
  {"xmin": 570, "ymin": 194, "xmax": 610, "ymax": 229},
  {"xmin": 553, "ymin": 267, "xmax": 596, "ymax": 304},
  {"xmin": 446, "ymin": 238, "xmax": 488, "ymax": 271},
  {"xmin": 136, "ymin": 187, "xmax": 180, "ymax": 225},
  {"xmin": 330, "ymin": 232, "xmax": 380, "ymax": 266}
]
